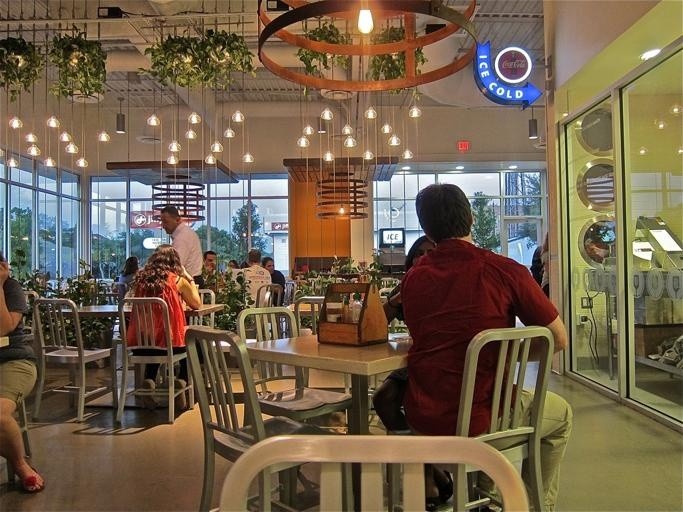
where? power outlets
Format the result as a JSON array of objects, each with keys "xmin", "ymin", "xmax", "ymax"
[{"xmin": 576, "ymin": 314, "xmax": 590, "ymax": 326}]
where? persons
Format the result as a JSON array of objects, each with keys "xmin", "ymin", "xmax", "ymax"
[
  {"xmin": 118, "ymin": 256, "xmax": 141, "ymax": 294},
  {"xmin": 402, "ymin": 184, "xmax": 573, "ymax": 512},
  {"xmin": 262, "ymin": 257, "xmax": 285, "ymax": 307},
  {"xmin": 530, "ymin": 246, "xmax": 543, "ymax": 285},
  {"xmin": 228, "ymin": 260, "xmax": 239, "ymax": 269},
  {"xmin": 241, "ymin": 262, "xmax": 250, "ymax": 269},
  {"xmin": 0, "ymin": 249, "xmax": 47, "ymax": 494},
  {"xmin": 241, "ymin": 249, "xmax": 271, "ymax": 308},
  {"xmin": 127, "ymin": 244, "xmax": 201, "ymax": 411},
  {"xmin": 161, "ymin": 207, "xmax": 203, "ymax": 296},
  {"xmin": 540, "ymin": 231, "xmax": 550, "ymax": 299},
  {"xmin": 383, "ymin": 235, "xmax": 453, "ymax": 511},
  {"xmin": 202, "ymin": 251, "xmax": 217, "ymax": 279}
]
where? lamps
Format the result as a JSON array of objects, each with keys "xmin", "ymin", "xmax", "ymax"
[
  {"xmin": 188, "ymin": 84, "xmax": 200, "ymax": 126},
  {"xmin": 24, "ymin": 79, "xmax": 40, "ymax": 145},
  {"xmin": 362, "ymin": 91, "xmax": 375, "ymax": 161},
  {"xmin": 45, "ymin": 64, "xmax": 59, "ymax": 131},
  {"xmin": 382, "ymin": 91, "xmax": 394, "ymax": 135},
  {"xmin": 43, "ymin": 127, "xmax": 57, "ymax": 167},
  {"xmin": 409, "ymin": 88, "xmax": 421, "ymax": 119},
  {"xmin": 7, "ymin": 130, "xmax": 20, "ymax": 170},
  {"xmin": 355, "ymin": 0, "xmax": 376, "ymax": 37},
  {"xmin": 319, "ymin": 87, "xmax": 334, "ymax": 124},
  {"xmin": 211, "ymin": 84, "xmax": 224, "ymax": 153},
  {"xmin": 240, "ymin": 99, "xmax": 255, "ymax": 164},
  {"xmin": 185, "ymin": 80, "xmax": 197, "ymax": 140},
  {"xmin": 402, "ymin": 92, "xmax": 414, "ymax": 161},
  {"xmin": 114, "ymin": 97, "xmax": 126, "ymax": 133},
  {"xmin": 168, "ymin": 29, "xmax": 182, "ymax": 151},
  {"xmin": 148, "ymin": 27, "xmax": 161, "ymax": 129},
  {"xmin": 529, "ymin": 105, "xmax": 543, "ymax": 140},
  {"xmin": 345, "ymin": 92, "xmax": 358, "ymax": 148},
  {"xmin": 303, "ymin": 88, "xmax": 315, "ymax": 136},
  {"xmin": 232, "ymin": 72, "xmax": 244, "ymax": 123},
  {"xmin": 76, "ymin": 102, "xmax": 90, "ymax": 170},
  {"xmin": 297, "ymin": 84, "xmax": 309, "ymax": 149},
  {"xmin": 338, "ymin": 205, "xmax": 347, "ymax": 217},
  {"xmin": 66, "ymin": 101, "xmax": 79, "ymax": 154},
  {"xmin": 59, "ymin": 99, "xmax": 73, "ymax": 142},
  {"xmin": 224, "ymin": 70, "xmax": 236, "ymax": 140},
  {"xmin": 365, "ymin": 92, "xmax": 377, "ymax": 121},
  {"xmin": 386, "ymin": 92, "xmax": 401, "ymax": 147},
  {"xmin": 204, "ymin": 90, "xmax": 217, "ymax": 164},
  {"xmin": 66, "ymin": 89, "xmax": 108, "ymax": 105},
  {"xmin": 96, "ymin": 93, "xmax": 110, "ymax": 142},
  {"xmin": 8, "ymin": 91, "xmax": 22, "ymax": 130},
  {"xmin": 26, "ymin": 141, "xmax": 42, "ymax": 157},
  {"xmin": 167, "ymin": 79, "xmax": 180, "ymax": 165},
  {"xmin": 341, "ymin": 92, "xmax": 351, "ymax": 136},
  {"xmin": 322, "ymin": 121, "xmax": 334, "ymax": 166}
]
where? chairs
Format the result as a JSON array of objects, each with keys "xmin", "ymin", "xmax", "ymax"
[
  {"xmin": 237, "ymin": 307, "xmax": 353, "ymax": 468},
  {"xmin": 380, "ymin": 326, "xmax": 554, "ymax": 512},
  {"xmin": 182, "ymin": 288, "xmax": 215, "ymax": 350},
  {"xmin": 222, "ymin": 284, "xmax": 285, "ymax": 337},
  {"xmin": 23, "ymin": 289, "xmax": 36, "ymax": 321},
  {"xmin": 111, "ymin": 298, "xmax": 197, "ymax": 426},
  {"xmin": 183, "ymin": 324, "xmax": 344, "ymax": 512},
  {"xmin": 217, "ymin": 435, "xmax": 530, "ymax": 512},
  {"xmin": 318, "ymin": 282, "xmax": 370, "ymax": 344},
  {"xmin": 33, "ymin": 298, "xmax": 116, "ymax": 422}
]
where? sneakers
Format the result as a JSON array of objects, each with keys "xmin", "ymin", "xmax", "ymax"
[
  {"xmin": 174, "ymin": 379, "xmax": 188, "ymax": 409},
  {"xmin": 142, "ymin": 379, "xmax": 161, "ymax": 409}
]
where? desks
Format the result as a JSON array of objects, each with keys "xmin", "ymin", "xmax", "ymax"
[
  {"xmin": 46, "ymin": 301, "xmax": 223, "ymax": 373},
  {"xmin": 294, "ymin": 296, "xmax": 390, "ymax": 335},
  {"xmin": 232, "ymin": 329, "xmax": 421, "ymax": 435}
]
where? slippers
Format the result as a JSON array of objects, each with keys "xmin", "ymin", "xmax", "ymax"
[{"xmin": 20, "ymin": 466, "xmax": 45, "ymax": 493}]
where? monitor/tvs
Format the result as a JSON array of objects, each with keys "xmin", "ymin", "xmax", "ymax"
[{"xmin": 379, "ymin": 228, "xmax": 406, "ymax": 249}]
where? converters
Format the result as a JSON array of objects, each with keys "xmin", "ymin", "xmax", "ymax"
[{"xmin": 581, "ymin": 314, "xmax": 589, "ymax": 322}]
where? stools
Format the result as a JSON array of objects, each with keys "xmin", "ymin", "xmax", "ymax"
[{"xmin": 0, "ymin": 398, "xmax": 32, "ymax": 486}]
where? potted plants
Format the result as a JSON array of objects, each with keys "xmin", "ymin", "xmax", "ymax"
[
  {"xmin": 301, "ymin": 24, "xmax": 350, "ymax": 71},
  {"xmin": 141, "ymin": 35, "xmax": 202, "ymax": 86},
  {"xmin": 76, "ymin": 259, "xmax": 113, "ymax": 349},
  {"xmin": 202, "ymin": 31, "xmax": 251, "ymax": 90},
  {"xmin": 50, "ymin": 24, "xmax": 108, "ymax": 97},
  {"xmin": 368, "ymin": 27, "xmax": 423, "ymax": 95},
  {"xmin": 0, "ymin": 33, "xmax": 42, "ymax": 102}
]
